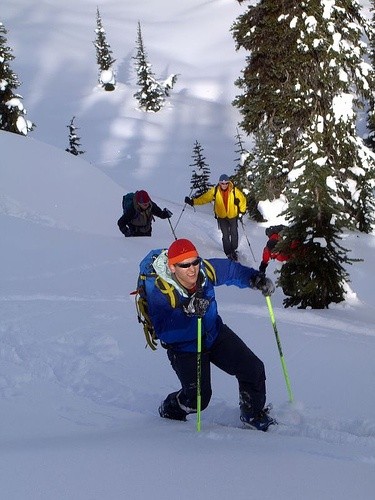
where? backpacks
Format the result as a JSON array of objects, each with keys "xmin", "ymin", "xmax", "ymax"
[
  {"xmin": 122, "ymin": 193, "xmax": 136, "ymax": 214},
  {"xmin": 130, "ymin": 248, "xmax": 216, "ymax": 350}
]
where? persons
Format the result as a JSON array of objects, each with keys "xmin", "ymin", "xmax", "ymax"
[
  {"xmin": 145, "ymin": 238, "xmax": 279, "ymax": 432},
  {"xmin": 259, "ymin": 233, "xmax": 308, "ymax": 274},
  {"xmin": 117, "ymin": 189, "xmax": 172, "ymax": 237},
  {"xmin": 185, "ymin": 174, "xmax": 246, "ymax": 261}
]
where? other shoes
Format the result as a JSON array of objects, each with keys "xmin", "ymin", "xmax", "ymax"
[
  {"xmin": 157, "ymin": 403, "xmax": 170, "ymax": 420},
  {"xmin": 228, "ymin": 257, "xmax": 232, "ymax": 260},
  {"xmin": 232, "ymin": 251, "xmax": 237, "ymax": 262},
  {"xmin": 240, "ymin": 410, "xmax": 277, "ymax": 432}
]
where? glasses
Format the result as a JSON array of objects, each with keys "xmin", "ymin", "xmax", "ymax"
[{"xmin": 177, "ymin": 259, "xmax": 200, "ymax": 269}]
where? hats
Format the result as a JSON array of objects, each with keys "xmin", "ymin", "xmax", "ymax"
[
  {"xmin": 137, "ymin": 190, "xmax": 150, "ymax": 203},
  {"xmin": 219, "ymin": 174, "xmax": 229, "ymax": 184},
  {"xmin": 168, "ymin": 238, "xmax": 198, "ymax": 265}
]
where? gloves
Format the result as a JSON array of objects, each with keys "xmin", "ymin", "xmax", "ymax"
[
  {"xmin": 251, "ymin": 274, "xmax": 276, "ymax": 297},
  {"xmin": 185, "ymin": 197, "xmax": 190, "ymax": 204},
  {"xmin": 163, "ymin": 208, "xmax": 173, "ymax": 218},
  {"xmin": 259, "ymin": 261, "xmax": 268, "ymax": 271},
  {"xmin": 183, "ymin": 292, "xmax": 210, "ymax": 317}
]
